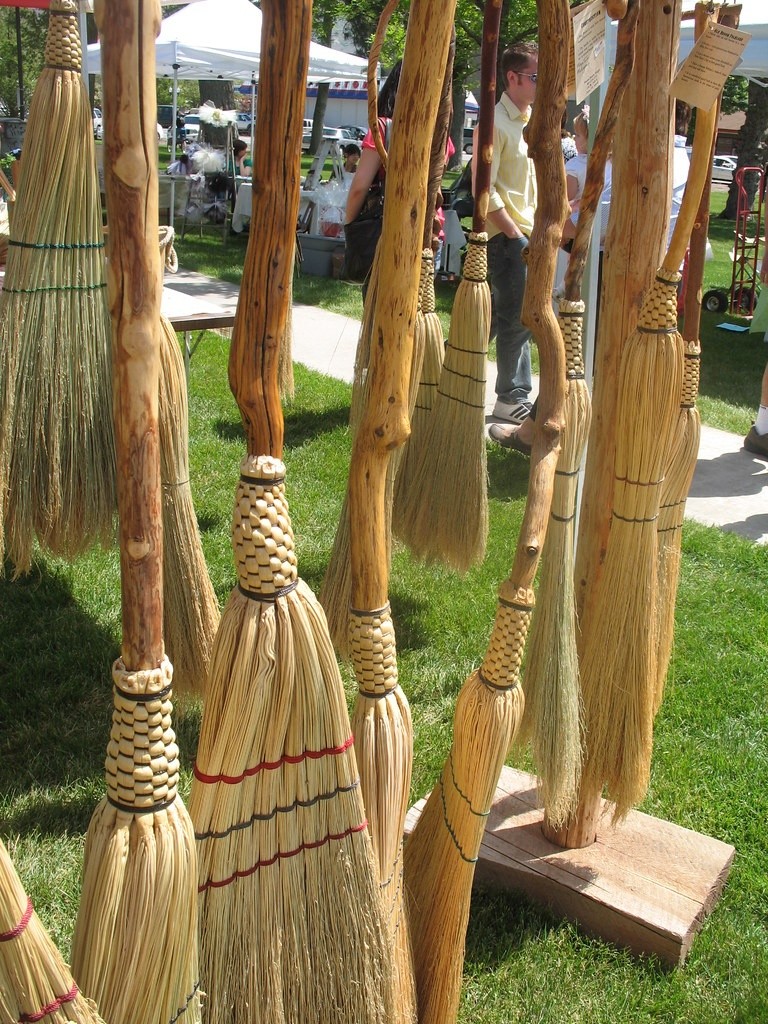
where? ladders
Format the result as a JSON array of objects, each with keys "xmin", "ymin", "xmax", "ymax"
[
  {"xmin": 302, "ymin": 135, "xmax": 349, "ymax": 191},
  {"xmin": 180, "ymin": 118, "xmax": 243, "ymax": 247}
]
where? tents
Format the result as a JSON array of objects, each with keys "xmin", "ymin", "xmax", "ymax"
[
  {"xmin": 577, "ymin": 0, "xmax": 768, "ymax": 396},
  {"xmin": 0, "ymin": 0, "xmax": 381, "ymax": 227}
]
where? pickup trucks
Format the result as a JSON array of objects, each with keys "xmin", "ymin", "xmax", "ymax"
[{"xmin": 302, "ymin": 119, "xmax": 325, "ymax": 132}]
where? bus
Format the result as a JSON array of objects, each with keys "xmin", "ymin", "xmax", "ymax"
[{"xmin": 157, "ymin": 105, "xmax": 180, "ymax": 141}]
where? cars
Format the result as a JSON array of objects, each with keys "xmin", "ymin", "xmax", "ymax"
[
  {"xmin": 167, "ymin": 114, "xmax": 204, "ymax": 145},
  {"xmin": 711, "ymin": 155, "xmax": 738, "ymax": 182},
  {"xmin": 463, "ymin": 128, "xmax": 473, "ymax": 154},
  {"xmin": 338, "ymin": 125, "xmax": 369, "ymax": 140},
  {"xmin": 0, "ymin": 117, "xmax": 26, "ymax": 162},
  {"xmin": 301, "ymin": 127, "xmax": 363, "ymax": 154}
]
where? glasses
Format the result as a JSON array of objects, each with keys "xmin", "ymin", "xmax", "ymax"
[{"xmin": 517, "ymin": 72, "xmax": 537, "ymax": 82}]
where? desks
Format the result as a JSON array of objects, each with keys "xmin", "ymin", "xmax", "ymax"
[
  {"xmin": 158, "ymin": 173, "xmax": 193, "ymax": 225},
  {"xmin": 232, "ymin": 182, "xmax": 318, "ymax": 260}
]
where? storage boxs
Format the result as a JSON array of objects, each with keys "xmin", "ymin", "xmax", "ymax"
[{"xmin": 294, "ymin": 232, "xmax": 347, "ymax": 277}]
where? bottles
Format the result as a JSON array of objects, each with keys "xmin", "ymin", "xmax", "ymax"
[{"xmin": 333, "ymin": 244, "xmax": 346, "ymax": 281}]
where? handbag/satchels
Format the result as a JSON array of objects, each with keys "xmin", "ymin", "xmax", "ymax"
[{"xmin": 345, "ymin": 183, "xmax": 383, "ymax": 283}]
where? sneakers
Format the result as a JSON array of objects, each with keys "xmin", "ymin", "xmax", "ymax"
[{"xmin": 492, "ymin": 398, "xmax": 534, "ymax": 424}]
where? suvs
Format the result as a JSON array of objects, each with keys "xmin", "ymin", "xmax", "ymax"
[
  {"xmin": 237, "ymin": 113, "xmax": 256, "ymax": 135},
  {"xmin": 93, "ymin": 108, "xmax": 102, "ymax": 139}
]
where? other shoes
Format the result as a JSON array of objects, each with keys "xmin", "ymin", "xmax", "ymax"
[
  {"xmin": 488, "ymin": 422, "xmax": 530, "ymax": 454},
  {"xmin": 743, "ymin": 425, "xmax": 768, "ymax": 458}
]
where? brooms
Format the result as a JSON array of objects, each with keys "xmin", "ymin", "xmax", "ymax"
[{"xmin": 0, "ymin": 5, "xmax": 746, "ymax": 1021}]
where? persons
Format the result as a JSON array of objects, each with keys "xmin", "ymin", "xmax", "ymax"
[
  {"xmin": 344, "ymin": 57, "xmax": 456, "ymax": 377},
  {"xmin": 473, "ymin": 40, "xmax": 536, "ymax": 425},
  {"xmin": 745, "ymin": 190, "xmax": 768, "ymax": 458},
  {"xmin": 490, "ymin": 96, "xmax": 689, "ymax": 458},
  {"xmin": 328, "ymin": 144, "xmax": 361, "ymax": 181},
  {"xmin": 223, "ymin": 140, "xmax": 252, "ymax": 237}
]
